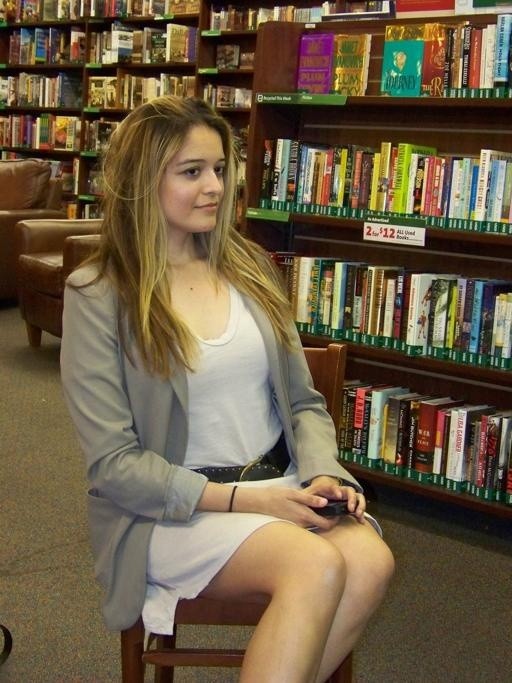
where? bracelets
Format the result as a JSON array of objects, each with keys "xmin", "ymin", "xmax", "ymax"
[{"xmin": 227, "ymin": 485, "xmax": 238, "ymax": 513}]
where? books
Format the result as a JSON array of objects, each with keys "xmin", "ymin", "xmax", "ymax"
[
  {"xmin": 0, "ymin": 0, "xmax": 202, "ymax": 220},
  {"xmin": 205, "ymin": 0, "xmax": 511, "ymax": 506}
]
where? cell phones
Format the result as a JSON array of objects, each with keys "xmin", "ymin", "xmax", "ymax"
[{"xmin": 308, "ymin": 498, "xmax": 360, "ymax": 516}]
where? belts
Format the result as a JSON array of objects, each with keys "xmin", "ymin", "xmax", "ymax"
[{"xmin": 188, "ymin": 428, "xmax": 293, "ymax": 484}]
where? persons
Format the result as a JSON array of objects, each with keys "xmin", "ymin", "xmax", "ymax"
[{"xmin": 59, "ymin": 92, "xmax": 396, "ymax": 683}]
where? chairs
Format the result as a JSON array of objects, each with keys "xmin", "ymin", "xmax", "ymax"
[{"xmin": 120, "ymin": 343, "xmax": 353, "ymax": 683}]
